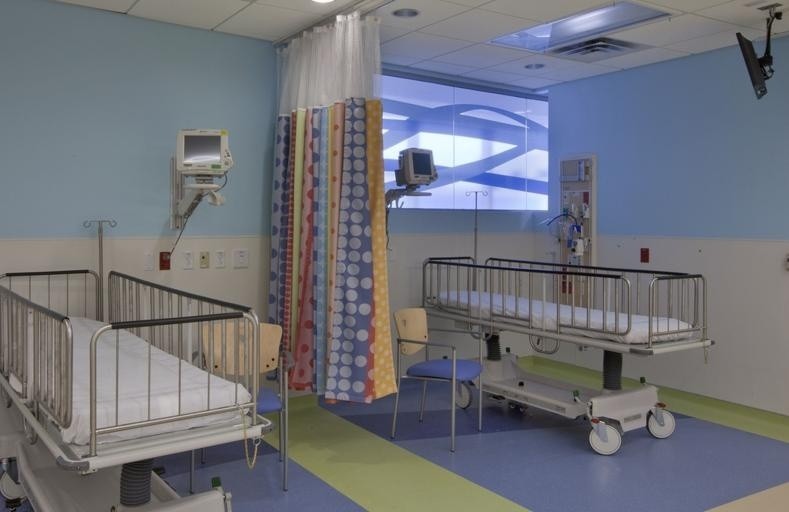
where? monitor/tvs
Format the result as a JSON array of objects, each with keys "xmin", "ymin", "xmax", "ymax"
[
  {"xmin": 734, "ymin": 32, "xmax": 767, "ymax": 100},
  {"xmin": 177, "ymin": 129, "xmax": 233, "ymax": 173},
  {"xmin": 396, "ymin": 148, "xmax": 438, "ymax": 184}
]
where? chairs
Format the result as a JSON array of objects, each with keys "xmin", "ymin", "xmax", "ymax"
[
  {"xmin": 187, "ymin": 320, "xmax": 298, "ymax": 495},
  {"xmin": 390, "ymin": 307, "xmax": 485, "ymax": 454}
]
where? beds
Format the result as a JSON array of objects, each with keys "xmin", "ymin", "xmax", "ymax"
[
  {"xmin": 421, "ymin": 255, "xmax": 715, "ymax": 456},
  {"xmin": 1, "ymin": 268, "xmax": 275, "ymax": 512}
]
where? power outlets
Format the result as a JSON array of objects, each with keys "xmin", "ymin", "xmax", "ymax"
[{"xmin": 159, "ymin": 252, "xmax": 171, "ymax": 270}]
